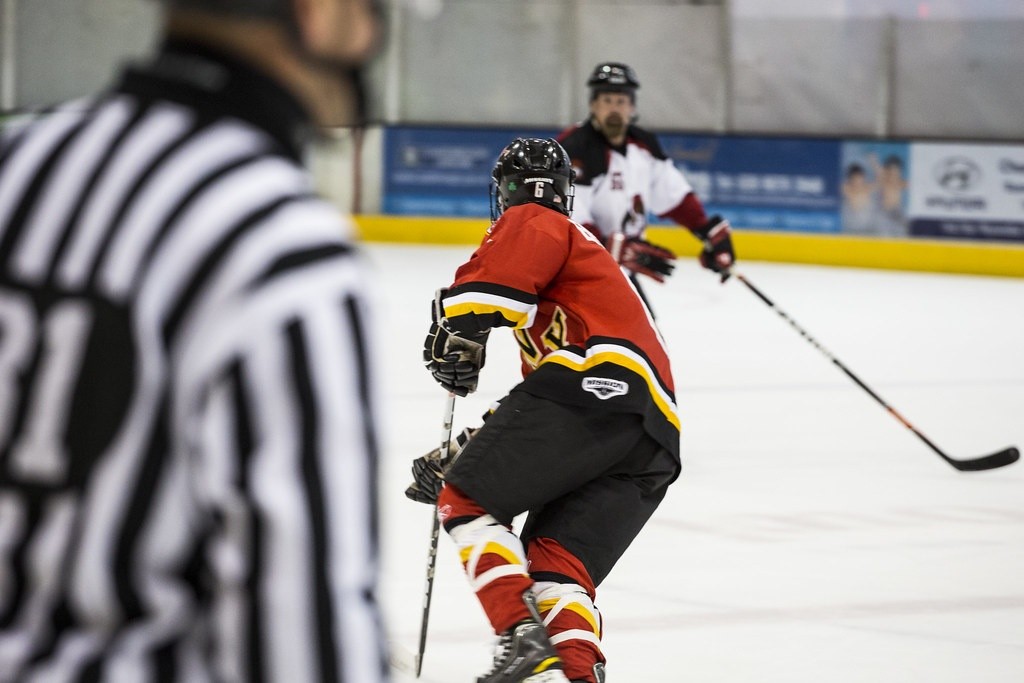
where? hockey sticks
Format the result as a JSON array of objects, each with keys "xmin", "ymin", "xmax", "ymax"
[
  {"xmin": 697, "ymin": 248, "xmax": 1020, "ymax": 471},
  {"xmin": 415, "ymin": 350, "xmax": 459, "ymax": 680}
]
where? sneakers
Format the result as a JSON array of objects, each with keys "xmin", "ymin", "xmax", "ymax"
[{"xmin": 475, "ymin": 588, "xmax": 571, "ymax": 683}]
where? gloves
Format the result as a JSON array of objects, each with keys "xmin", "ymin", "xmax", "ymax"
[
  {"xmin": 605, "ymin": 231, "xmax": 678, "ymax": 284},
  {"xmin": 691, "ymin": 214, "xmax": 737, "ymax": 284},
  {"xmin": 404, "ymin": 395, "xmax": 507, "ymax": 506},
  {"xmin": 423, "ymin": 287, "xmax": 492, "ymax": 398}
]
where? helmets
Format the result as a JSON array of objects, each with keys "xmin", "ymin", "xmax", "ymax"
[
  {"xmin": 585, "ymin": 61, "xmax": 641, "ymax": 89},
  {"xmin": 488, "ymin": 137, "xmax": 577, "ymax": 224}
]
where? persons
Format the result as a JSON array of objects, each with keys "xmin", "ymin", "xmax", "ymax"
[
  {"xmin": 556, "ymin": 64, "xmax": 735, "ymax": 281},
  {"xmin": 404, "ymin": 136, "xmax": 682, "ymax": 683},
  {"xmin": 846, "ymin": 154, "xmax": 907, "ymax": 215},
  {"xmin": 0, "ymin": 0, "xmax": 393, "ymax": 683}
]
